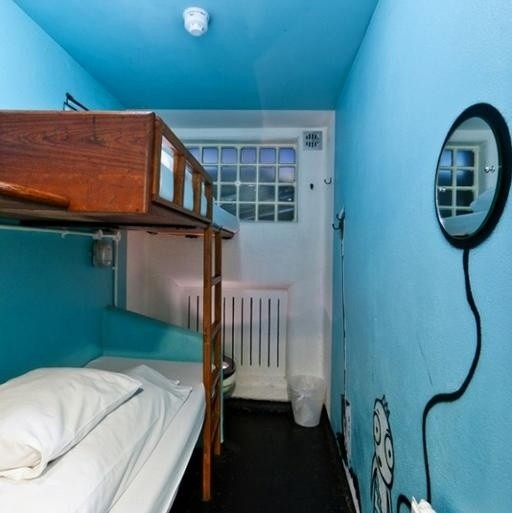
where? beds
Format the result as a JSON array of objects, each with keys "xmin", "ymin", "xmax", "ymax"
[
  {"xmin": 0, "ymin": 109, "xmax": 240, "ymax": 512},
  {"xmin": 441, "ymin": 210, "xmax": 490, "ymax": 238}
]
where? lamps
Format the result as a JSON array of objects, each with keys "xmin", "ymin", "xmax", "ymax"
[{"xmin": 183, "ymin": 6, "xmax": 210, "ymax": 37}]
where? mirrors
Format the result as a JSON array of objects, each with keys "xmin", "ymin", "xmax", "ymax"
[{"xmin": 434, "ymin": 102, "xmax": 512, "ymax": 249}]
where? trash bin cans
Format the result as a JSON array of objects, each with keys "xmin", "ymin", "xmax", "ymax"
[{"xmin": 288, "ymin": 375, "xmax": 327, "ymax": 427}]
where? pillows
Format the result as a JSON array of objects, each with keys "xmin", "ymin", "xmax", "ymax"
[
  {"xmin": 0, "ymin": 366, "xmax": 143, "ymax": 481},
  {"xmin": 469, "ymin": 187, "xmax": 496, "ymax": 212}
]
